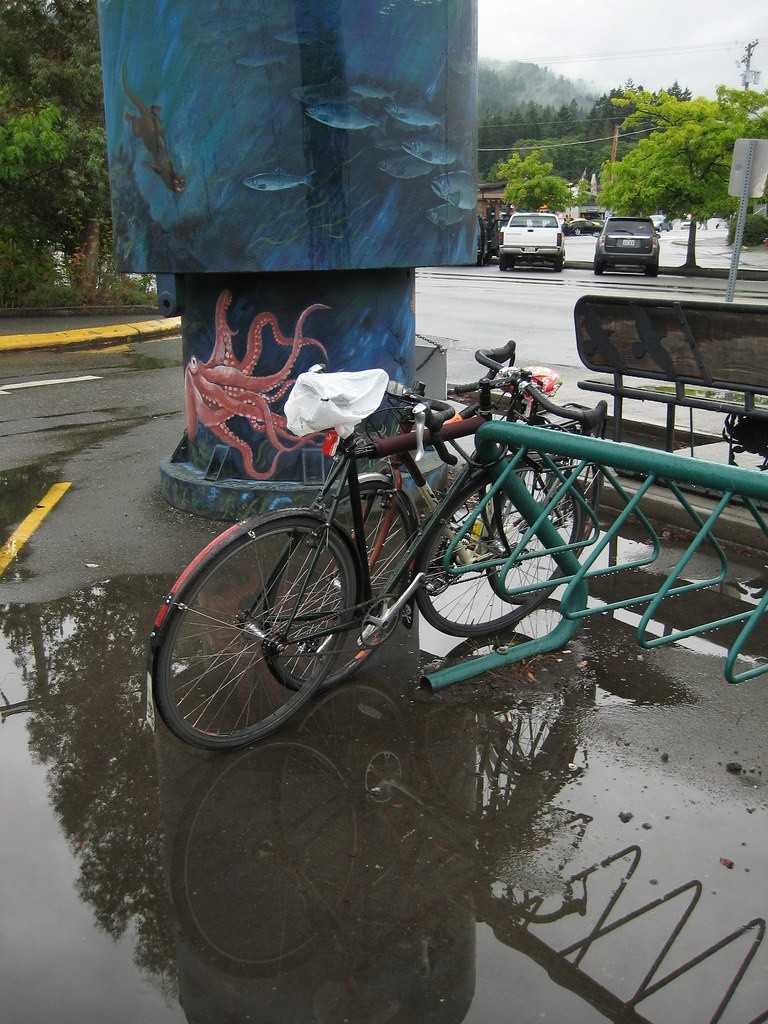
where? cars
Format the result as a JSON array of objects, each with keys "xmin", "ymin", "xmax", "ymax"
[
  {"xmin": 564, "ymin": 215, "xmax": 605, "ymax": 236},
  {"xmin": 679, "ymin": 215, "xmax": 708, "ymax": 230},
  {"xmin": 649, "ymin": 215, "xmax": 673, "ymax": 232}
]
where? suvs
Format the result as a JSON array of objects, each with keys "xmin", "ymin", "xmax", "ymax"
[{"xmin": 592, "ymin": 216, "xmax": 660, "ymax": 278}]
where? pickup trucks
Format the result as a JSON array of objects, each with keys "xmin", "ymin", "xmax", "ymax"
[{"xmin": 488, "ymin": 212, "xmax": 566, "ymax": 273}]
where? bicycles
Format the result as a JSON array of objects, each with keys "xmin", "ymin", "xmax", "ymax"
[{"xmin": 140, "ymin": 339, "xmax": 604, "ymax": 752}]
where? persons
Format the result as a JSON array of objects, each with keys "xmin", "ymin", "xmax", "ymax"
[{"xmin": 564, "ymin": 215, "xmax": 573, "ymax": 223}]
